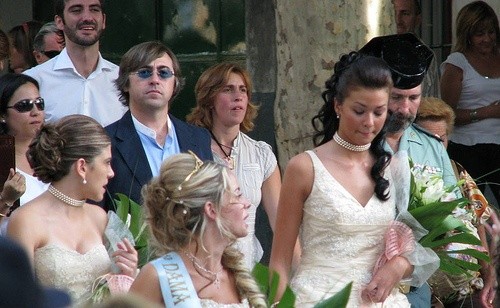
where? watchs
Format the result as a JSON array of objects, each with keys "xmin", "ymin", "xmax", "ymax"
[{"xmin": 470, "ymin": 109, "xmax": 479, "ymax": 122}]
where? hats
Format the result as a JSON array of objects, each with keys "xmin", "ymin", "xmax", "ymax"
[{"xmin": 357, "ymin": 32, "xmax": 434, "ymax": 89}]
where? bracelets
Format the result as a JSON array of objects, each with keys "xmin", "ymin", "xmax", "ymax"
[{"xmin": 0, "ymin": 213, "xmax": 5, "ymax": 217}]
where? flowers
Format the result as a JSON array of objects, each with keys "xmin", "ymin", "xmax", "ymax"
[
  {"xmin": 407, "ymin": 160, "xmax": 491, "ymax": 296},
  {"xmin": 114, "ymin": 193, "xmax": 149, "ymax": 267}
]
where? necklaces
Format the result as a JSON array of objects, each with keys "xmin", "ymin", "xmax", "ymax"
[
  {"xmin": 185, "ymin": 250, "xmax": 223, "ymax": 288},
  {"xmin": 208, "ymin": 127, "xmax": 237, "ymax": 169},
  {"xmin": 48, "ymin": 185, "xmax": 86, "ymax": 207},
  {"xmin": 333, "ymin": 131, "xmax": 371, "ymax": 151}
]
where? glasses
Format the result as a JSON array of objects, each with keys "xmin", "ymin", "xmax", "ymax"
[
  {"xmin": 41, "ymin": 50, "xmax": 60, "ymax": 59},
  {"xmin": 9, "ymin": 98, "xmax": 44, "ymax": 113},
  {"xmin": 132, "ymin": 68, "xmax": 174, "ymax": 80}
]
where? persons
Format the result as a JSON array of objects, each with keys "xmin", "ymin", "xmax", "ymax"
[
  {"xmin": 357, "ymin": 33, "xmax": 483, "ymax": 308},
  {"xmin": 185, "ymin": 62, "xmax": 303, "ymax": 279},
  {"xmin": 1, "ymin": 20, "xmax": 65, "ymax": 76},
  {"xmin": 439, "ymin": 0, "xmax": 500, "ymax": 262},
  {"xmin": 269, "ymin": 51, "xmax": 439, "ymax": 308},
  {"xmin": 19, "ymin": 0, "xmax": 130, "ymax": 129},
  {"xmin": 86, "ymin": 41, "xmax": 213, "ymax": 216},
  {"xmin": 0, "ymin": 74, "xmax": 55, "ymax": 221},
  {"xmin": 125, "ymin": 149, "xmax": 268, "ymax": 308},
  {"xmin": 6, "ymin": 114, "xmax": 141, "ymax": 308},
  {"xmin": 414, "ymin": 94, "xmax": 500, "ymax": 308},
  {"xmin": 0, "ymin": 235, "xmax": 70, "ymax": 308}
]
